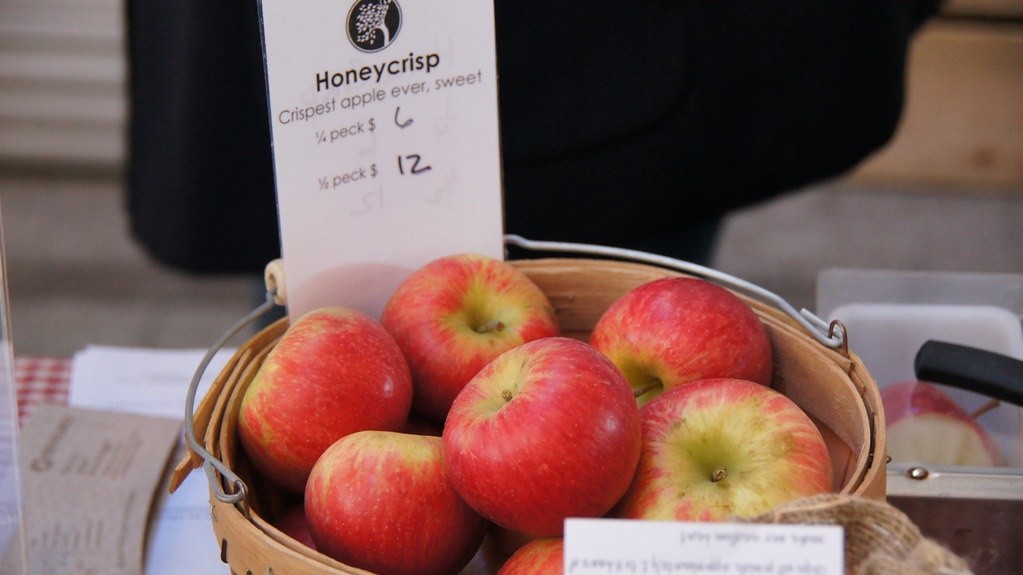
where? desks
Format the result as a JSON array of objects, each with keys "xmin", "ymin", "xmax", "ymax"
[{"xmin": 0, "ymin": 354, "xmax": 1012, "ymax": 575}]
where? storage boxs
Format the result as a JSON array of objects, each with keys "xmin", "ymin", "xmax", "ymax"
[{"xmin": 830, "ymin": 304, "xmax": 1022, "ymax": 471}]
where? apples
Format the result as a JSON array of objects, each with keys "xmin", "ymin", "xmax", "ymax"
[
  {"xmin": 236, "ymin": 253, "xmax": 833, "ymax": 575},
  {"xmin": 878, "ymin": 378, "xmax": 1008, "ymax": 469}
]
